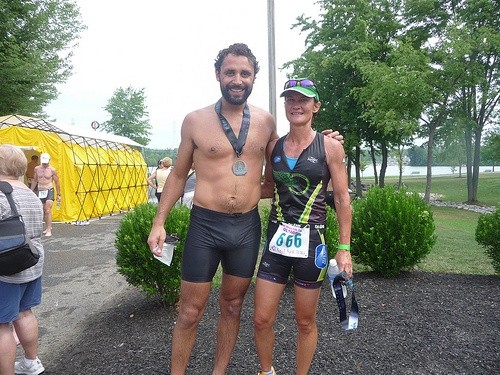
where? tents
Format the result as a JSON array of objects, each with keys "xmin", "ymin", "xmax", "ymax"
[{"xmin": 1, "ymin": 112, "xmax": 151, "ymax": 226}]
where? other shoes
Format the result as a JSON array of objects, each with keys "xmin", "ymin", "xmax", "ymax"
[{"xmin": 258, "ymin": 365, "xmax": 277, "ymax": 375}]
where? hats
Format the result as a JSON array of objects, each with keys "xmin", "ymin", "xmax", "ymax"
[
  {"xmin": 40, "ymin": 153, "xmax": 51, "ymax": 163},
  {"xmin": 280, "ymin": 78, "xmax": 319, "ymax": 101}
]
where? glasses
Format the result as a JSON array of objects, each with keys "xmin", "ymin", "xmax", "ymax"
[{"xmin": 284, "ymin": 79, "xmax": 319, "ymax": 95}]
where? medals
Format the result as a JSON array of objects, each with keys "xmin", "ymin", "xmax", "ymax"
[{"xmin": 232, "ymin": 159, "xmax": 249, "ymax": 177}]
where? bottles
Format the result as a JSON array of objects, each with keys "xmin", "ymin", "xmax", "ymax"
[
  {"xmin": 56, "ymin": 199, "xmax": 60, "ymax": 210},
  {"xmin": 327, "ymin": 259, "xmax": 347, "ymax": 298}
]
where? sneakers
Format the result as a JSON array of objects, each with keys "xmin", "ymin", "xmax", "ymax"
[{"xmin": 15, "ymin": 356, "xmax": 44, "ymax": 375}]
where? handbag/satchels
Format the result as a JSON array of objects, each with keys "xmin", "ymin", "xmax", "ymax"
[{"xmin": 0, "ymin": 181, "xmax": 40, "ymax": 276}]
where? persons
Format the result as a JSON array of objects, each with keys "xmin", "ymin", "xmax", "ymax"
[
  {"xmin": 255, "ymin": 77, "xmax": 354, "ymax": 375},
  {"xmin": 147, "ymin": 156, "xmax": 179, "ymax": 207},
  {"xmin": 1, "ymin": 146, "xmax": 62, "ymax": 375},
  {"xmin": 147, "ymin": 43, "xmax": 346, "ymax": 375}
]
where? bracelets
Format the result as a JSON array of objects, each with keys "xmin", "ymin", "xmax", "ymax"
[
  {"xmin": 57, "ymin": 192, "xmax": 61, "ymax": 195},
  {"xmin": 338, "ymin": 244, "xmax": 350, "ymax": 251}
]
